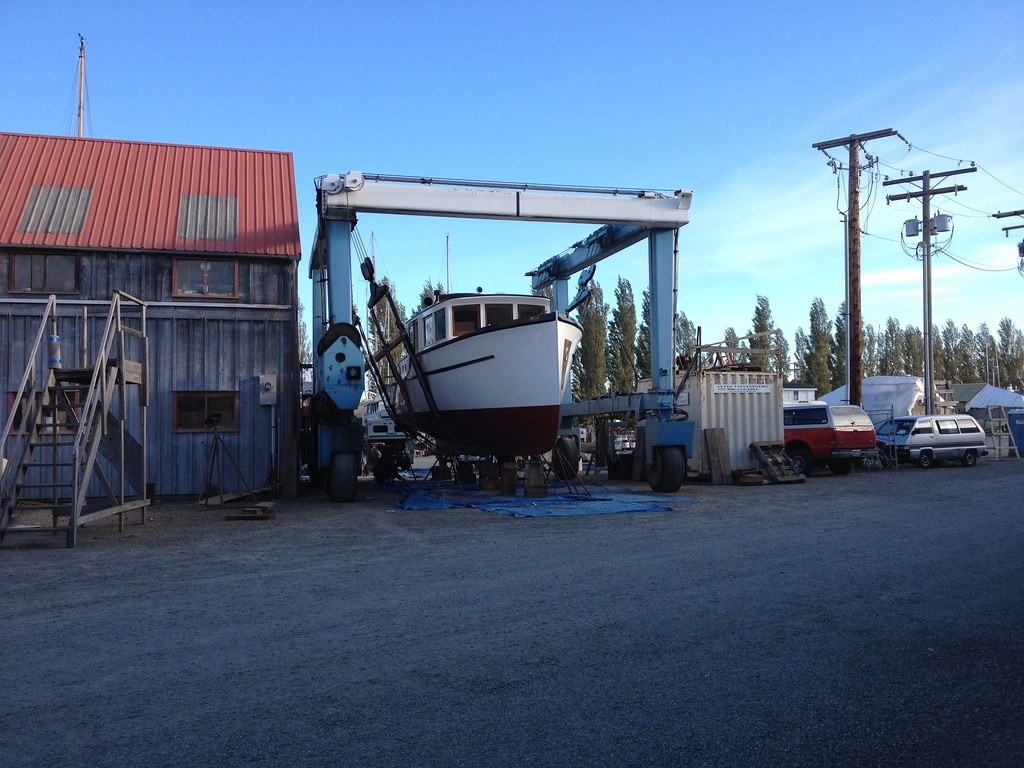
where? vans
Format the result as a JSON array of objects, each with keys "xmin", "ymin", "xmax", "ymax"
[
  {"xmin": 875, "ymin": 414, "xmax": 990, "ymax": 468},
  {"xmin": 782, "ymin": 403, "xmax": 878, "ymax": 476}
]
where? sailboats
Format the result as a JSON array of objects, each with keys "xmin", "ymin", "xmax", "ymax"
[{"xmin": 355, "ymin": 231, "xmax": 417, "ymax": 458}]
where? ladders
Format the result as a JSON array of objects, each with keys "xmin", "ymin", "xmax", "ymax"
[{"xmin": 983, "ymin": 406, "xmax": 1020, "ymax": 461}]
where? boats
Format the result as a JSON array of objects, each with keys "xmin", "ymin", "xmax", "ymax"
[{"xmin": 389, "ymin": 227, "xmax": 587, "ymax": 460}]
